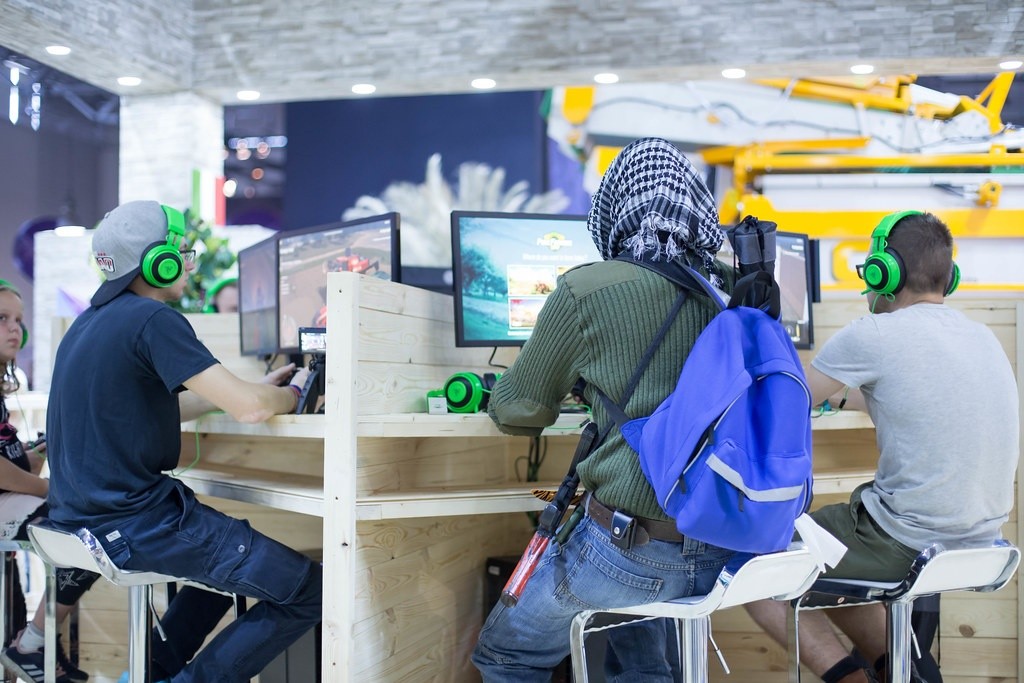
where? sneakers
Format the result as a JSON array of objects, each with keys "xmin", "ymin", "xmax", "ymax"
[{"xmin": 0, "ymin": 628, "xmax": 88, "ymax": 682}]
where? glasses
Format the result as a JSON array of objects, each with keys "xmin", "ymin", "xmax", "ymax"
[
  {"xmin": 179, "ymin": 247, "xmax": 196, "ymax": 265},
  {"xmin": 856, "ymin": 263, "xmax": 866, "ymax": 279}
]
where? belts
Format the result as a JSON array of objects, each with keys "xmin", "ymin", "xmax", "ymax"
[{"xmin": 578, "ymin": 491, "xmax": 683, "ymax": 549}]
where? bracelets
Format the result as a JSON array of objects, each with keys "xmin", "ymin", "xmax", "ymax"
[{"xmin": 289, "ymin": 383, "xmax": 302, "ymax": 413}]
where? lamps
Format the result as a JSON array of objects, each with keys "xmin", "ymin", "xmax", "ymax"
[
  {"xmin": 1000, "ymin": 61, "xmax": 1023, "ymax": 70},
  {"xmin": 46, "ymin": 46, "xmax": 71, "ymax": 56},
  {"xmin": 594, "ymin": 73, "xmax": 619, "ymax": 84},
  {"xmin": 117, "ymin": 77, "xmax": 142, "ymax": 86},
  {"xmin": 2, "ymin": 54, "xmax": 43, "ymax": 131},
  {"xmin": 471, "ymin": 79, "xmax": 496, "ymax": 89},
  {"xmin": 851, "ymin": 64, "xmax": 874, "ymax": 75},
  {"xmin": 352, "ymin": 84, "xmax": 376, "ymax": 95},
  {"xmin": 237, "ymin": 90, "xmax": 260, "ymax": 101},
  {"xmin": 722, "ymin": 68, "xmax": 746, "ymax": 79}
]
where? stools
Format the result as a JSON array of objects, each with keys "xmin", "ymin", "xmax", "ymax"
[
  {"xmin": 26, "ymin": 516, "xmax": 259, "ymax": 683},
  {"xmin": 0, "ymin": 540, "xmax": 80, "ymax": 683},
  {"xmin": 569, "ymin": 542, "xmax": 824, "ymax": 683},
  {"xmin": 786, "ymin": 538, "xmax": 1022, "ymax": 682}
]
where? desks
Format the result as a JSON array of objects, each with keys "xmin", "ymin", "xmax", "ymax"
[{"xmin": 13, "ymin": 287, "xmax": 1022, "ymax": 683}]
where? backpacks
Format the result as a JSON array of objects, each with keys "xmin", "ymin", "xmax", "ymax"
[{"xmin": 587, "ymin": 248, "xmax": 812, "ymax": 553}]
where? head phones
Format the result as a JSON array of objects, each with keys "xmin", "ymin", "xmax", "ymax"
[
  {"xmin": 0, "ymin": 278, "xmax": 28, "ymax": 350},
  {"xmin": 140, "ymin": 205, "xmax": 186, "ymax": 288},
  {"xmin": 862, "ymin": 210, "xmax": 960, "ymax": 298},
  {"xmin": 426, "ymin": 371, "xmax": 502, "ymax": 414}
]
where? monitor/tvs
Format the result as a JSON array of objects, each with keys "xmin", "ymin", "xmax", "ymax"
[
  {"xmin": 715, "ymin": 224, "xmax": 815, "ymax": 351},
  {"xmin": 237, "ymin": 211, "xmax": 401, "ymax": 357},
  {"xmin": 448, "ymin": 209, "xmax": 606, "ymax": 348}
]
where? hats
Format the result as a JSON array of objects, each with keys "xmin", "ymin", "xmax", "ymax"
[{"xmin": 85, "ymin": 200, "xmax": 168, "ymax": 308}]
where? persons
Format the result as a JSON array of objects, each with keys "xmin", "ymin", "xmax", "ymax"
[
  {"xmin": 471, "ymin": 138, "xmax": 811, "ymax": 683},
  {"xmin": 2, "ymin": 278, "xmax": 101, "ymax": 683},
  {"xmin": 49, "ymin": 202, "xmax": 322, "ymax": 683},
  {"xmin": 202, "ymin": 277, "xmax": 238, "ymax": 314},
  {"xmin": 742, "ymin": 209, "xmax": 1018, "ymax": 683}
]
